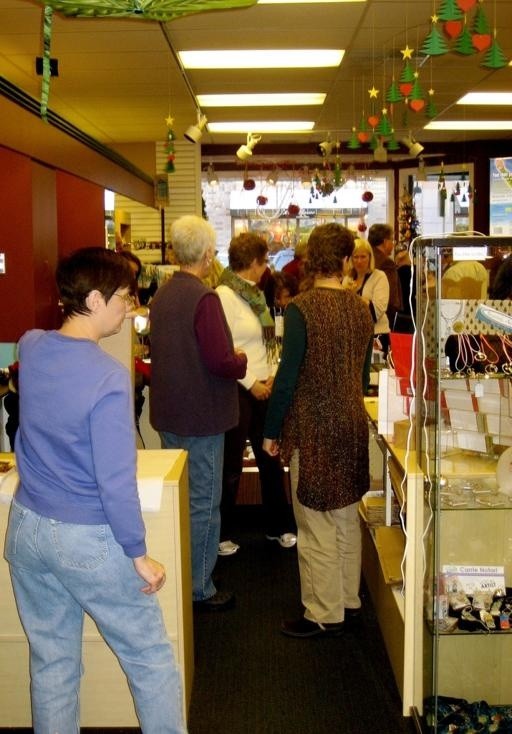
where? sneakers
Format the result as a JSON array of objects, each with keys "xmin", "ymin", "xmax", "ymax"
[
  {"xmin": 280, "ymin": 616, "xmax": 345, "ymax": 638},
  {"xmin": 218, "ymin": 539, "xmax": 240, "ymax": 556},
  {"xmin": 266, "ymin": 533, "xmax": 297, "ymax": 548}
]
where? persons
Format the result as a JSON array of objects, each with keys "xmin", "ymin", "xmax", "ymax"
[
  {"xmin": 6, "ymin": 246, "xmax": 187, "ymax": 734},
  {"xmin": 116, "ymin": 222, "xmax": 511, "ymax": 427},
  {"xmin": 147, "ymin": 212, "xmax": 249, "ymax": 614},
  {"xmin": 259, "ymin": 223, "xmax": 377, "ymax": 641},
  {"xmin": 209, "ymin": 232, "xmax": 300, "ymax": 557}
]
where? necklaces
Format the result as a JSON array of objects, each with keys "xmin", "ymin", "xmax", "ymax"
[{"xmin": 450, "ymin": 325, "xmax": 511, "ymax": 379}]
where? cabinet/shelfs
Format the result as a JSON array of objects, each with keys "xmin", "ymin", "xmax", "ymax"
[
  {"xmin": 360, "ymin": 394, "xmax": 511, "ymax": 705},
  {"xmin": 3, "ymin": 446, "xmax": 195, "ymax": 726},
  {"xmin": 412, "ymin": 234, "xmax": 512, "ymax": 730}
]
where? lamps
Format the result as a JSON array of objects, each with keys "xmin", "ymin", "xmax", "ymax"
[{"xmin": 182, "ymin": 114, "xmax": 469, "ymax": 205}]
